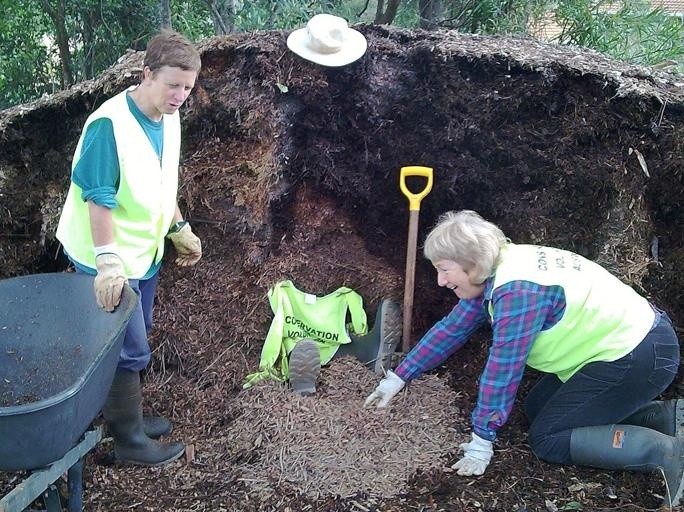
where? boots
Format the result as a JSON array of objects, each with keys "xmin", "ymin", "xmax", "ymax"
[
  {"xmin": 332, "ymin": 298, "xmax": 403, "ymax": 376},
  {"xmin": 288, "ymin": 338, "xmax": 321, "ymax": 394},
  {"xmin": 102, "ymin": 366, "xmax": 185, "ymax": 467},
  {"xmin": 570, "ymin": 426, "xmax": 684, "ymax": 506},
  {"xmin": 618, "ymin": 398, "xmax": 684, "ymax": 437}
]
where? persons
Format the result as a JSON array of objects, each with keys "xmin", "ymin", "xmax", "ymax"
[
  {"xmin": 363, "ymin": 209, "xmax": 684, "ymax": 508},
  {"xmin": 54, "ymin": 32, "xmax": 204, "ymax": 466}
]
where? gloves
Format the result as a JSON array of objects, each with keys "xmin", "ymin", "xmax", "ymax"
[
  {"xmin": 167, "ymin": 222, "xmax": 202, "ymax": 266},
  {"xmin": 94, "ymin": 253, "xmax": 128, "ymax": 311},
  {"xmin": 451, "ymin": 432, "xmax": 493, "ymax": 476},
  {"xmin": 364, "ymin": 369, "xmax": 405, "ymax": 408}
]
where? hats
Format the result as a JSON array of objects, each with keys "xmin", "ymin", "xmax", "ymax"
[{"xmin": 286, "ymin": 14, "xmax": 367, "ymax": 66}]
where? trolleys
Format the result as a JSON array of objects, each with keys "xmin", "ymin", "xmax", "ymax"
[{"xmin": 0, "ymin": 273, "xmax": 139, "ymax": 512}]
